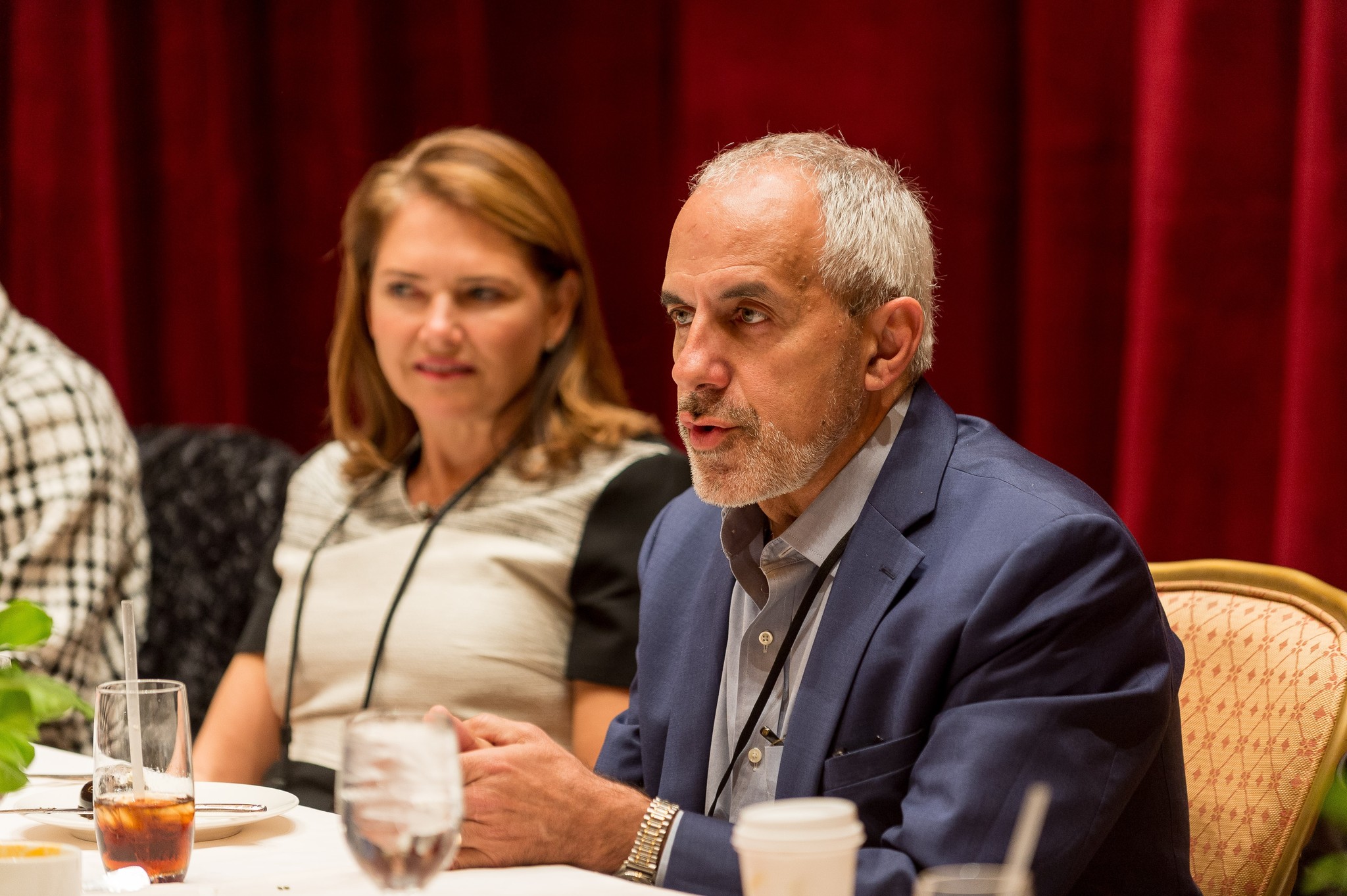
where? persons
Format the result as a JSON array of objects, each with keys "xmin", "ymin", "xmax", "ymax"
[
  {"xmin": 192, "ymin": 127, "xmax": 689, "ymax": 816},
  {"xmin": 450, "ymin": 130, "xmax": 1203, "ymax": 894},
  {"xmin": 1, "ymin": 285, "xmax": 153, "ymax": 758}
]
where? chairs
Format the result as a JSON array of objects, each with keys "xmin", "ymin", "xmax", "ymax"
[
  {"xmin": 123, "ymin": 418, "xmax": 306, "ymax": 790},
  {"xmin": 1150, "ymin": 555, "xmax": 1347, "ymax": 896}
]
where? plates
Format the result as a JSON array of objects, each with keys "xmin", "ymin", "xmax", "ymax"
[{"xmin": 15, "ymin": 781, "xmax": 299, "ymax": 842}]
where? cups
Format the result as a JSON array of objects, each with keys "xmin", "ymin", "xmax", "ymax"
[
  {"xmin": 0, "ymin": 842, "xmax": 82, "ymax": 896},
  {"xmin": 93, "ymin": 679, "xmax": 195, "ymax": 884},
  {"xmin": 729, "ymin": 795, "xmax": 867, "ymax": 896},
  {"xmin": 342, "ymin": 711, "xmax": 464, "ymax": 889},
  {"xmin": 911, "ymin": 863, "xmax": 1034, "ymax": 896}
]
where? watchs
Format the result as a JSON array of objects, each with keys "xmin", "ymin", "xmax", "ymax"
[{"xmin": 617, "ymin": 796, "xmax": 681, "ymax": 885}]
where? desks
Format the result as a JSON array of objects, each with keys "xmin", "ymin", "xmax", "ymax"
[{"xmin": 0, "ymin": 735, "xmax": 704, "ymax": 896}]
